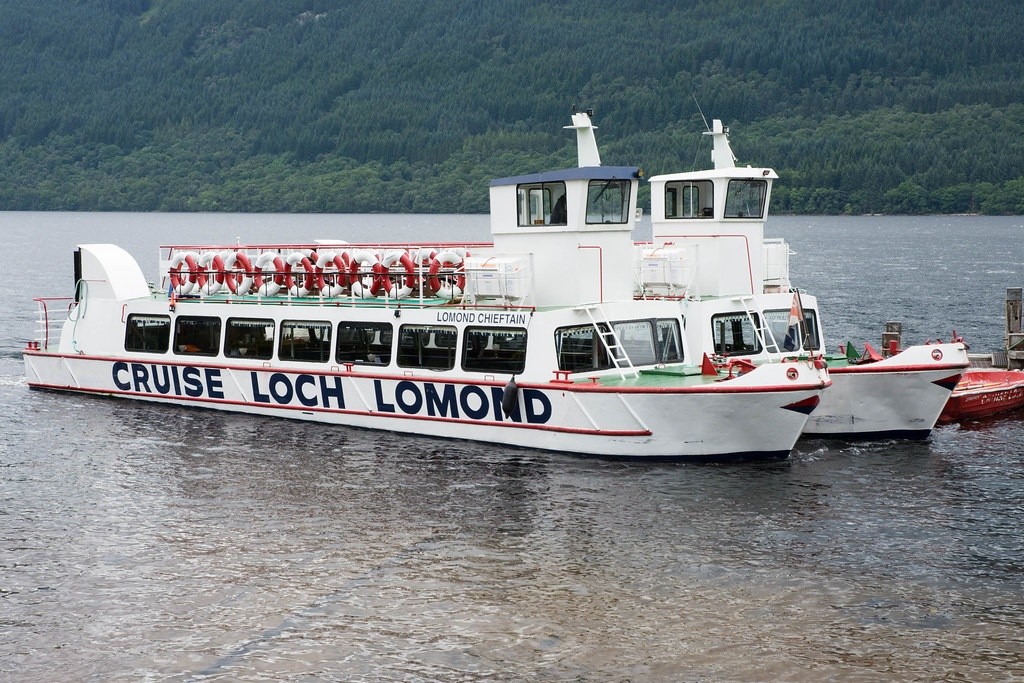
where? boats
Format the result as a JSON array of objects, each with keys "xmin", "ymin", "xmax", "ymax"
[
  {"xmin": 22, "ymin": 107, "xmax": 831, "ymax": 466},
  {"xmin": 940, "ymin": 372, "xmax": 1024, "ymax": 419},
  {"xmin": 646, "ymin": 118, "xmax": 971, "ymax": 443}
]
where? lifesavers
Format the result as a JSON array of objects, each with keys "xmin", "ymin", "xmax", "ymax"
[
  {"xmin": 169, "ymin": 253, "xmax": 197, "ymax": 294},
  {"xmin": 315, "ymin": 252, "xmax": 347, "ymax": 298},
  {"xmin": 301, "ymin": 248, "xmax": 472, "ymax": 267},
  {"xmin": 381, "ymin": 253, "xmax": 416, "ymax": 299},
  {"xmin": 197, "ymin": 252, "xmax": 224, "ymax": 295},
  {"xmin": 430, "ymin": 252, "xmax": 465, "ymax": 299},
  {"xmin": 284, "ymin": 252, "xmax": 314, "ymax": 297},
  {"xmin": 226, "ymin": 252, "xmax": 252, "ymax": 295},
  {"xmin": 349, "ymin": 252, "xmax": 381, "ymax": 300},
  {"xmin": 254, "ymin": 252, "xmax": 284, "ymax": 297}
]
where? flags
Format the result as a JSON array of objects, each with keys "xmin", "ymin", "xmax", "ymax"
[{"xmin": 787, "ymin": 287, "xmax": 806, "ymax": 326}]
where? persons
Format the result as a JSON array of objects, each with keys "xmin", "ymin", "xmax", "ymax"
[{"xmin": 550, "ymin": 193, "xmax": 567, "ymax": 224}]
